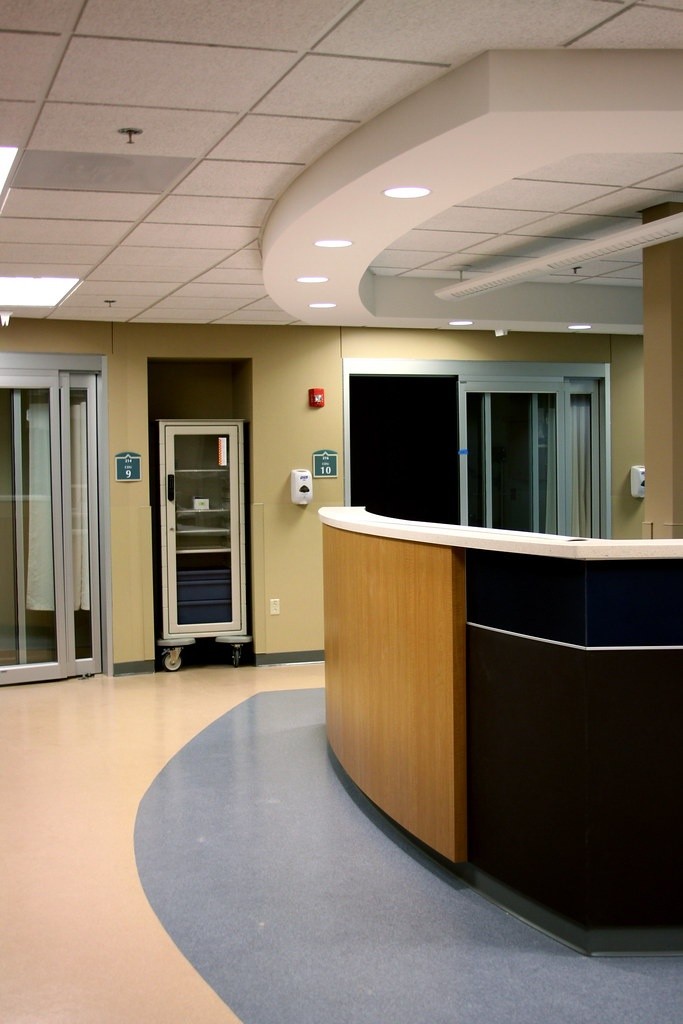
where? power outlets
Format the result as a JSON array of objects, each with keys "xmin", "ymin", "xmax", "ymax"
[{"xmin": 269, "ymin": 598, "xmax": 280, "ymax": 616}]
